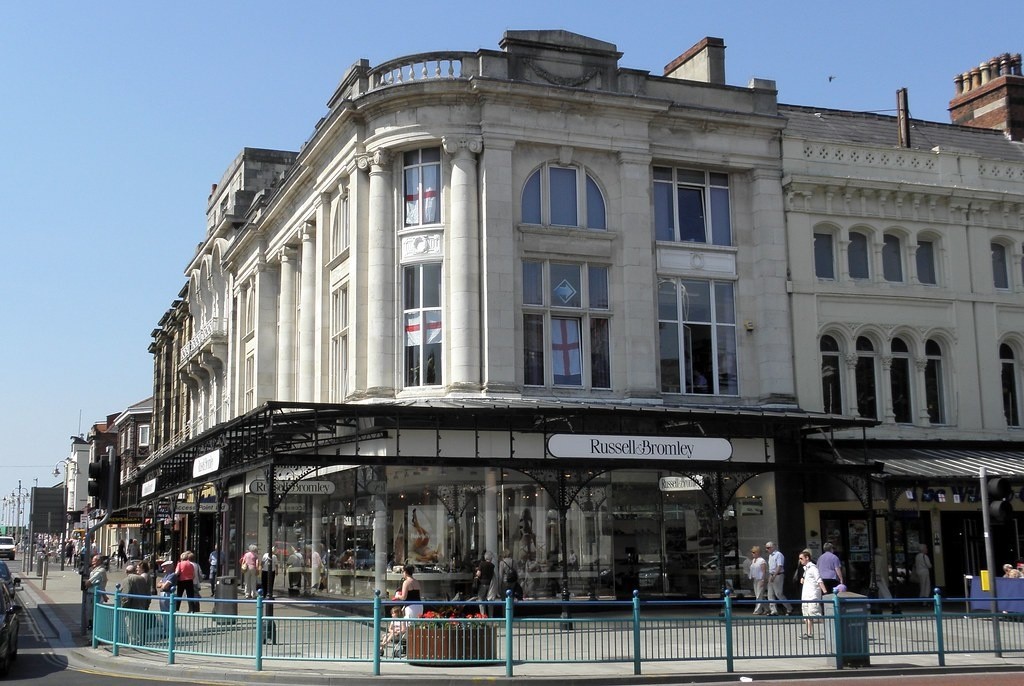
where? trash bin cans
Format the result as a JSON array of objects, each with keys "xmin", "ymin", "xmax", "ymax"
[
  {"xmin": 824, "ymin": 593, "xmax": 871, "ymax": 672},
  {"xmin": 36, "ymin": 554, "xmax": 48, "ymax": 577},
  {"xmin": 213, "ymin": 575, "xmax": 237, "ymax": 625}
]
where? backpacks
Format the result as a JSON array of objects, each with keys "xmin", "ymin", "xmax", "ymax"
[{"xmin": 501, "ymin": 560, "xmax": 518, "ymax": 583}]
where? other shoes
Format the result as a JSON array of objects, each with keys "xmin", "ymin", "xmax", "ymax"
[
  {"xmin": 767, "ymin": 612, "xmax": 779, "ymax": 616},
  {"xmin": 799, "ymin": 633, "xmax": 812, "ymax": 640},
  {"xmin": 763, "ymin": 608, "xmax": 770, "ymax": 615},
  {"xmin": 922, "ymin": 603, "xmax": 930, "ymax": 608},
  {"xmin": 786, "ymin": 608, "xmax": 793, "ymax": 615},
  {"xmin": 86, "ymin": 624, "xmax": 93, "ymax": 630},
  {"xmin": 752, "ymin": 611, "xmax": 764, "ymax": 615}
]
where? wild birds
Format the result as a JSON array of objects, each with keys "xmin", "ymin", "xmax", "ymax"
[{"xmin": 828, "ymin": 76, "xmax": 836, "ymax": 82}]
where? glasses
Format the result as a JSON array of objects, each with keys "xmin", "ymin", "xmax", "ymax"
[
  {"xmin": 752, "ymin": 552, "xmax": 757, "ymax": 554},
  {"xmin": 766, "ymin": 547, "xmax": 773, "ymax": 550},
  {"xmin": 799, "ymin": 558, "xmax": 807, "ymax": 560}
]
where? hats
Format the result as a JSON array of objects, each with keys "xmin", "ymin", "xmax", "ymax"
[{"xmin": 161, "ymin": 561, "xmax": 173, "ymax": 566}]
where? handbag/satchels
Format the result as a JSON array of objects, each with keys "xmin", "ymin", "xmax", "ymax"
[
  {"xmin": 241, "ymin": 554, "xmax": 248, "ymax": 574},
  {"xmin": 176, "ymin": 575, "xmax": 181, "ymax": 585},
  {"xmin": 910, "ymin": 567, "xmax": 920, "ymax": 582},
  {"xmin": 472, "ymin": 577, "xmax": 480, "ymax": 593}
]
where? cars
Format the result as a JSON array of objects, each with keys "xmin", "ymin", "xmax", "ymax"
[
  {"xmin": 600, "ymin": 569, "xmax": 614, "ymax": 590},
  {"xmin": 638, "ymin": 554, "xmax": 751, "ymax": 592},
  {"xmin": 0, "ymin": 560, "xmax": 21, "ymax": 601},
  {"xmin": 334, "ymin": 548, "xmax": 376, "ymax": 569},
  {"xmin": 274, "ymin": 541, "xmax": 290, "ymax": 561},
  {"xmin": 0, "ymin": 581, "xmax": 23, "ymax": 682}
]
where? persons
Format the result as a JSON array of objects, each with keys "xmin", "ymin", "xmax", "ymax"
[
  {"xmin": 117, "ymin": 539, "xmax": 141, "ymax": 567},
  {"xmin": 121, "ymin": 560, "xmax": 177, "ymax": 645},
  {"xmin": 85, "ymin": 555, "xmax": 108, "ymax": 643},
  {"xmin": 1003, "ymin": 564, "xmax": 1024, "ymax": 621},
  {"xmin": 11, "ymin": 532, "xmax": 97, "ymax": 567},
  {"xmin": 176, "ymin": 551, "xmax": 204, "ymax": 612},
  {"xmin": 916, "ymin": 544, "xmax": 931, "ymax": 607},
  {"xmin": 766, "ymin": 541, "xmax": 794, "ymax": 615},
  {"xmin": 497, "ymin": 547, "xmax": 582, "ymax": 597},
  {"xmin": 817, "ymin": 543, "xmax": 843, "ymax": 594},
  {"xmin": 277, "ymin": 542, "xmax": 397, "ymax": 593},
  {"xmin": 209, "ymin": 545, "xmax": 226, "ymax": 596},
  {"xmin": 500, "ymin": 550, "xmax": 518, "ymax": 617},
  {"xmin": 750, "ymin": 546, "xmax": 769, "ymax": 614},
  {"xmin": 239, "ymin": 544, "xmax": 279, "ymax": 601},
  {"xmin": 475, "ymin": 552, "xmax": 496, "ymax": 619},
  {"xmin": 797, "ymin": 549, "xmax": 827, "ymax": 639},
  {"xmin": 381, "ymin": 565, "xmax": 423, "ymax": 646}
]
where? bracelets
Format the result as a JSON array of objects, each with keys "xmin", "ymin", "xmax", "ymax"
[
  {"xmin": 762, "ymin": 579, "xmax": 764, "ymax": 581},
  {"xmin": 773, "ymin": 575, "xmax": 775, "ymax": 577}
]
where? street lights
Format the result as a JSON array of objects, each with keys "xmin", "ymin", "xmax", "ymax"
[
  {"xmin": 1, "ymin": 479, "xmax": 30, "ymax": 572},
  {"xmin": 52, "ymin": 457, "xmax": 82, "ymax": 572}
]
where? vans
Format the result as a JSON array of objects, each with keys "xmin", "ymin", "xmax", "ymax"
[{"xmin": 0, "ymin": 536, "xmax": 19, "ymax": 560}]
